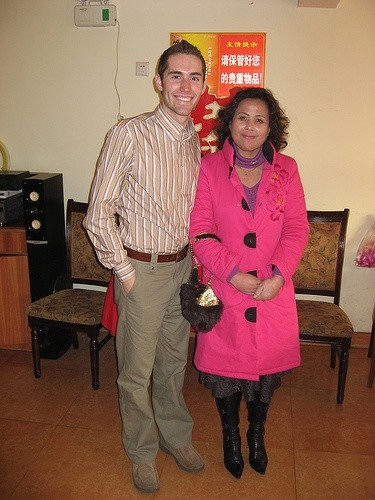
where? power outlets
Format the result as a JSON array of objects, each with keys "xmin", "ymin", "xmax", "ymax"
[{"xmin": 136, "ymin": 62, "xmax": 149, "ymax": 75}]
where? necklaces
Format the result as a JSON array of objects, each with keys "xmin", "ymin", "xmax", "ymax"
[{"xmin": 237, "ymin": 165, "xmax": 256, "ymax": 176}]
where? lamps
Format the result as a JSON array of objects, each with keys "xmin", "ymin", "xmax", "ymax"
[{"xmin": 75, "ymin": 0, "xmax": 118, "ymax": 27}]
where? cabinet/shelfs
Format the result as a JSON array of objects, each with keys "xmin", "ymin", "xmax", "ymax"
[{"xmin": 0, "ymin": 228, "xmax": 31, "ymax": 351}]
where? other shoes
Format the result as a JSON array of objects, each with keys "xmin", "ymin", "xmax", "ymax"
[
  {"xmin": 159, "ymin": 436, "xmax": 205, "ymax": 472},
  {"xmin": 132, "ymin": 462, "xmax": 161, "ymax": 493}
]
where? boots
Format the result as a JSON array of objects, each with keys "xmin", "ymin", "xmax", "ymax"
[
  {"xmin": 244, "ymin": 398, "xmax": 270, "ymax": 475},
  {"xmin": 215, "ymin": 391, "xmax": 245, "ymax": 480}
]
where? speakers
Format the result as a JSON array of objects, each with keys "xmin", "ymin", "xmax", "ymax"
[{"xmin": 20, "ymin": 173, "xmax": 76, "ymax": 360}]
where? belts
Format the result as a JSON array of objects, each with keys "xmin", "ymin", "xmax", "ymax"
[{"xmin": 122, "ymin": 241, "xmax": 191, "ymax": 262}]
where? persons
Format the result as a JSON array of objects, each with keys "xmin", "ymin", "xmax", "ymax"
[
  {"xmin": 189, "ymin": 87, "xmax": 309, "ymax": 478},
  {"xmin": 83, "ymin": 41, "xmax": 206, "ymax": 490}
]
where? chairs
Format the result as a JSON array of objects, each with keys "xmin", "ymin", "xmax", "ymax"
[
  {"xmin": 25, "ymin": 199, "xmax": 120, "ymax": 390},
  {"xmin": 291, "ymin": 208, "xmax": 355, "ymax": 404}
]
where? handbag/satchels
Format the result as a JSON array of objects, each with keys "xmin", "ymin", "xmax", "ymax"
[{"xmin": 179, "ymin": 233, "xmax": 224, "ymax": 334}]
